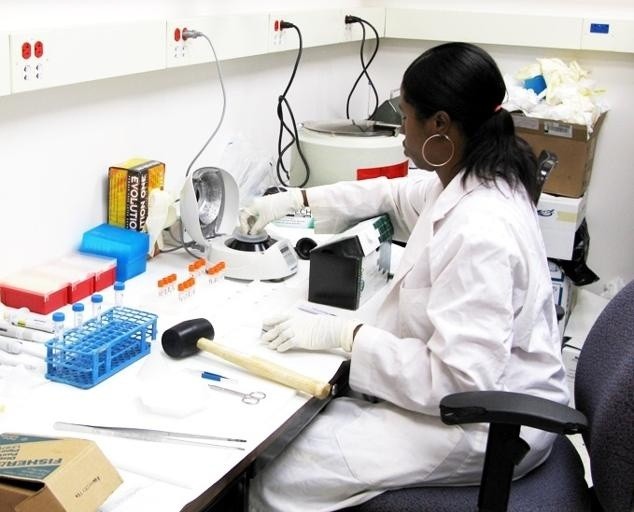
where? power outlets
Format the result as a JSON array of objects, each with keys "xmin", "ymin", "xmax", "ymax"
[
  {"xmin": 9, "ymin": 29, "xmax": 49, "ymax": 92},
  {"xmin": 165, "ymin": 18, "xmax": 192, "ymax": 65},
  {"xmin": 269, "ymin": 13, "xmax": 286, "ymax": 47},
  {"xmin": 340, "ymin": 12, "xmax": 354, "ymax": 38}
]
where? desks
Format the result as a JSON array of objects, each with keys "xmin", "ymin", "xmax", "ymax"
[{"xmin": 1, "ymin": 219, "xmax": 409, "ymax": 511}]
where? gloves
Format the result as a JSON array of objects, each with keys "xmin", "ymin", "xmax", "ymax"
[
  {"xmin": 261, "ymin": 311, "xmax": 364, "ymax": 355},
  {"xmin": 238, "ymin": 187, "xmax": 305, "ymax": 236}
]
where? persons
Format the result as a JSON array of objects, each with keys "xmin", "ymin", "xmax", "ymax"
[{"xmin": 237, "ymin": 39, "xmax": 572, "ymax": 512}]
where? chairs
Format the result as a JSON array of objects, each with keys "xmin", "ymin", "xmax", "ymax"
[{"xmin": 361, "ymin": 279, "xmax": 634, "ymax": 512}]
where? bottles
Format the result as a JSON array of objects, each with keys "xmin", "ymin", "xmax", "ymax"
[
  {"xmin": 71, "ymin": 304, "xmax": 84, "ymax": 339},
  {"xmin": 90, "ymin": 294, "xmax": 102, "ymax": 333},
  {"xmin": 52, "ymin": 313, "xmax": 65, "ymax": 374},
  {"xmin": 157, "ymin": 257, "xmax": 226, "ymax": 303},
  {"xmin": 113, "ymin": 283, "xmax": 124, "ymax": 310}
]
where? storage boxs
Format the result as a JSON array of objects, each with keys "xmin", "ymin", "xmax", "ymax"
[
  {"xmin": 561, "ymin": 340, "xmax": 581, "ymax": 411},
  {"xmin": 534, "ymin": 192, "xmax": 590, "ymax": 263},
  {"xmin": 1, "ymin": 433, "xmax": 123, "ymax": 512},
  {"xmin": 503, "ymin": 108, "xmax": 609, "ymax": 199},
  {"xmin": 549, "ymin": 279, "xmax": 574, "ymax": 349}
]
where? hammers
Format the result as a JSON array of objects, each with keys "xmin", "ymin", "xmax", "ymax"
[{"xmin": 161, "ymin": 318, "xmax": 330, "ymax": 401}]
